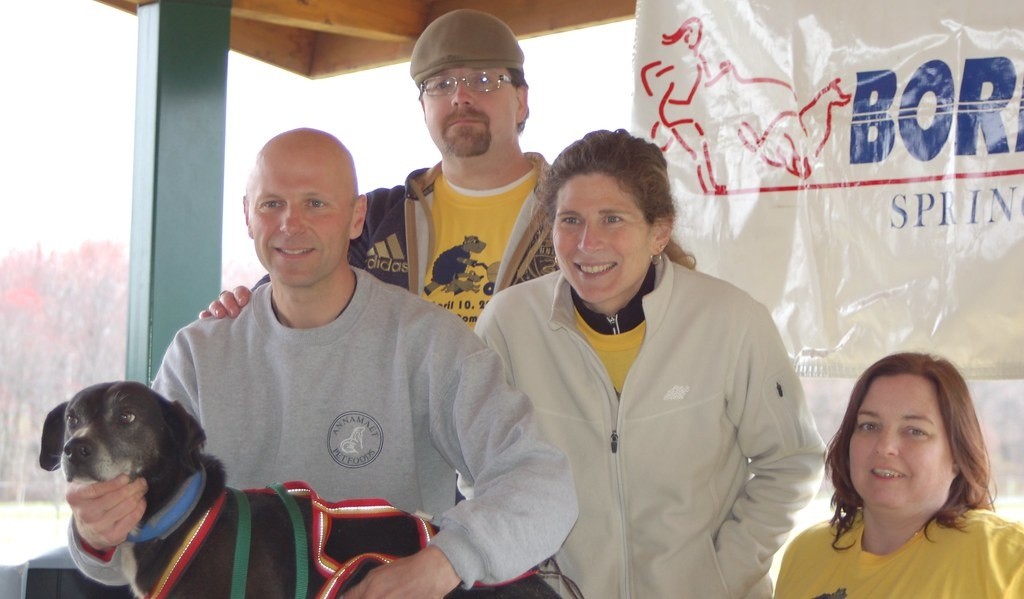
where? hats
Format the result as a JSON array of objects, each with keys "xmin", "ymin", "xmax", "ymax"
[{"xmin": 411, "ymin": 8, "xmax": 525, "ymax": 88}]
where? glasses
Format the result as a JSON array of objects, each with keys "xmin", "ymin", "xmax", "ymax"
[{"xmin": 422, "ymin": 73, "xmax": 513, "ymax": 94}]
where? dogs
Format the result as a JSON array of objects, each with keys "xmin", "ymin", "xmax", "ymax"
[{"xmin": 37, "ymin": 381, "xmax": 563, "ymax": 599}]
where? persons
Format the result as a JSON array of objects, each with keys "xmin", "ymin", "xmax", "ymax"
[
  {"xmin": 456, "ymin": 130, "xmax": 828, "ymax": 599},
  {"xmin": 777, "ymin": 352, "xmax": 1024, "ymax": 599},
  {"xmin": 199, "ymin": 10, "xmax": 695, "ymax": 333},
  {"xmin": 65, "ymin": 126, "xmax": 580, "ymax": 599}
]
